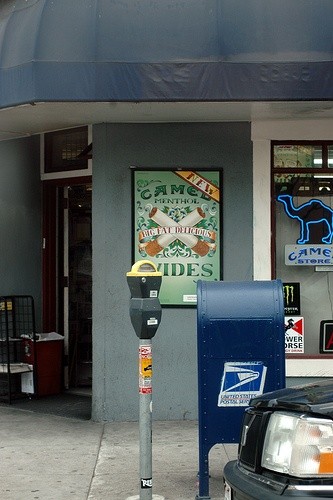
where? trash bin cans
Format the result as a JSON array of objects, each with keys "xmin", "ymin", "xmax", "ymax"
[{"xmin": 20, "ymin": 332, "xmax": 65, "ymax": 398}]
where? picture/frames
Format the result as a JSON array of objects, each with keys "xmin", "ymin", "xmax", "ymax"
[{"xmin": 130, "ymin": 165, "xmax": 225, "ymax": 310}]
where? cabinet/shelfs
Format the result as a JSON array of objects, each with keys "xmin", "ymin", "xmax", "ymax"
[
  {"xmin": 1, "ymin": 294, "xmax": 37, "ymax": 406},
  {"xmin": 20, "ymin": 332, "xmax": 65, "ymax": 401}
]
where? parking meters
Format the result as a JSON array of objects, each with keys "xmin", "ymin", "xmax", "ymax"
[{"xmin": 125, "ymin": 260, "xmax": 164, "ymax": 495}]
